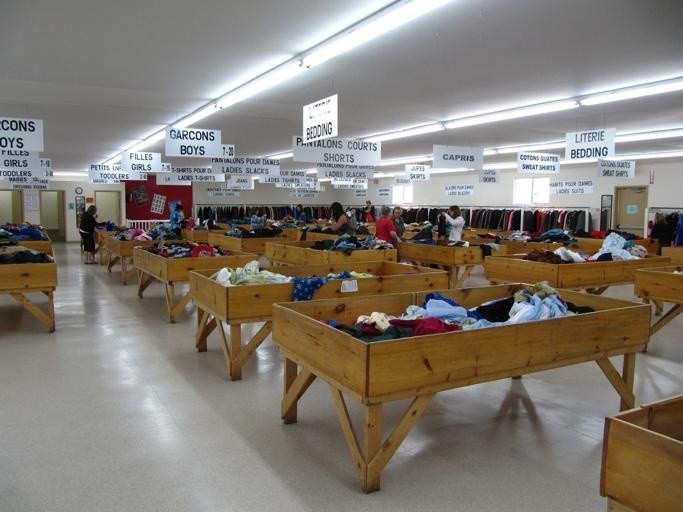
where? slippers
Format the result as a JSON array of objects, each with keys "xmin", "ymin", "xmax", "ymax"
[{"xmin": 84, "ymin": 262, "xmax": 99, "ymax": 264}]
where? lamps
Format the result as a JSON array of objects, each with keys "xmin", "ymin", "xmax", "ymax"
[{"xmin": 98, "ymin": 0, "xmax": 683, "ymax": 179}]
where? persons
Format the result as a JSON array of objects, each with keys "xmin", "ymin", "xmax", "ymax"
[
  {"xmin": 329, "ymin": 201, "xmax": 353, "ymax": 237},
  {"xmin": 294, "ymin": 204, "xmax": 307, "ymax": 226},
  {"xmin": 78, "ymin": 204, "xmax": 107, "ymax": 265},
  {"xmin": 375, "ymin": 204, "xmax": 395, "ymax": 242},
  {"xmin": 361, "ymin": 200, "xmax": 374, "ymax": 223},
  {"xmin": 435, "ymin": 205, "xmax": 467, "ymax": 242},
  {"xmin": 390, "ymin": 207, "xmax": 404, "ymax": 237},
  {"xmin": 168, "ymin": 204, "xmax": 185, "ymax": 241},
  {"xmin": 250, "ymin": 207, "xmax": 267, "ymax": 226},
  {"xmin": 649, "ymin": 212, "xmax": 674, "ymax": 255}
]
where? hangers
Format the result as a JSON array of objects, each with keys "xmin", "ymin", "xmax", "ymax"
[
  {"xmin": 194, "ymin": 202, "xmax": 591, "ymax": 222},
  {"xmin": 655, "ymin": 207, "xmax": 682, "ymax": 215}
]
